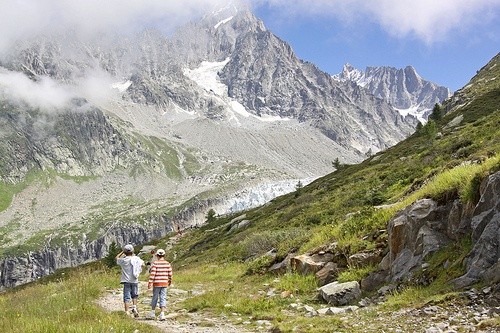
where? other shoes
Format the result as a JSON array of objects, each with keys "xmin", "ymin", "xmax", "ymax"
[
  {"xmin": 151, "ymin": 311, "xmax": 155, "ymax": 319},
  {"xmin": 159, "ymin": 312, "xmax": 165, "ymax": 320},
  {"xmin": 132, "ymin": 307, "xmax": 139, "ymax": 317}
]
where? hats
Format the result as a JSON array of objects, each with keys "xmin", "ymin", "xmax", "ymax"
[
  {"xmin": 155, "ymin": 248, "xmax": 165, "ymax": 256},
  {"xmin": 124, "ymin": 243, "xmax": 134, "ymax": 253}
]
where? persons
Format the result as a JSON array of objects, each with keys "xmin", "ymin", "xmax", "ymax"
[
  {"xmin": 114, "ymin": 244, "xmax": 148, "ymax": 318},
  {"xmin": 147, "ymin": 249, "xmax": 172, "ymax": 321}
]
